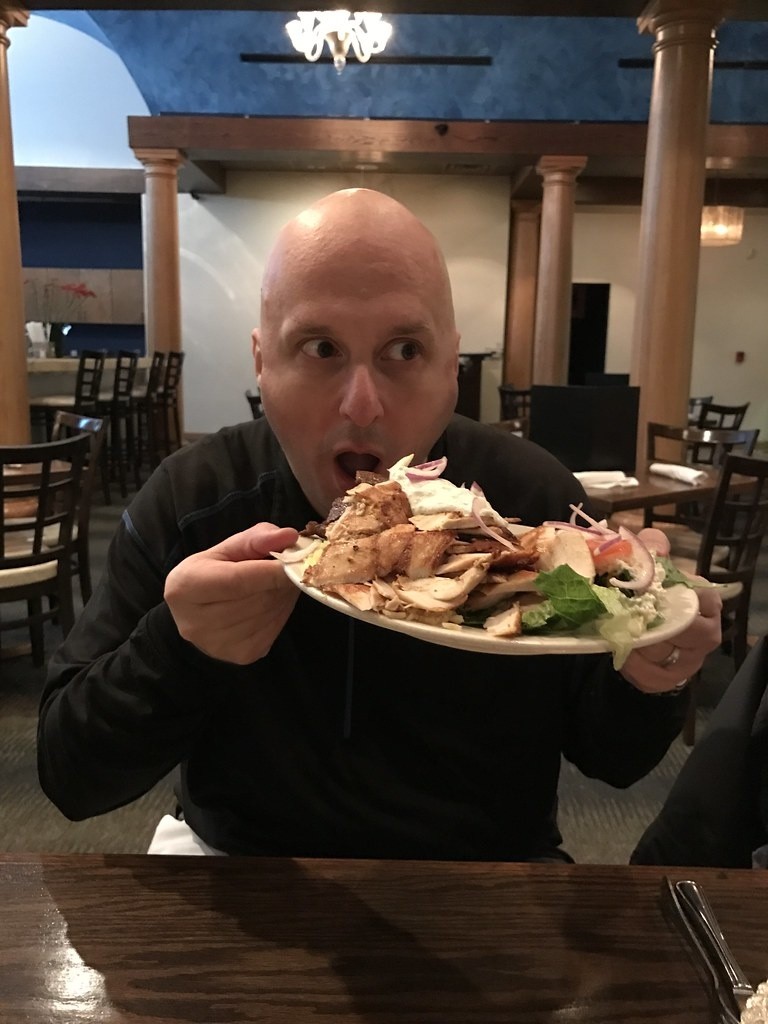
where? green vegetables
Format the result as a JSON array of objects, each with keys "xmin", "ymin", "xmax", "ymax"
[{"xmin": 516, "ymin": 554, "xmax": 730, "ymax": 670}]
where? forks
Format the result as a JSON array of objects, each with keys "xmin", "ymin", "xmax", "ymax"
[{"xmin": 659, "ymin": 874, "xmax": 740, "ymax": 1024}]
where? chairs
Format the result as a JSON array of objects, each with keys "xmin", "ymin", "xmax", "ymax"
[
  {"xmin": 607, "ymin": 422, "xmax": 761, "ymax": 653},
  {"xmin": 678, "ymin": 401, "xmax": 752, "ymax": 537},
  {"xmin": 0, "ymin": 431, "xmax": 94, "ymax": 668},
  {"xmin": 684, "ymin": 394, "xmax": 714, "ymax": 464},
  {"xmin": 668, "ymin": 452, "xmax": 768, "ymax": 746},
  {"xmin": 245, "ymin": 388, "xmax": 266, "ymax": 420},
  {"xmin": 4, "ymin": 410, "xmax": 109, "ymax": 627},
  {"xmin": 495, "ymin": 382, "xmax": 532, "ymax": 420}
]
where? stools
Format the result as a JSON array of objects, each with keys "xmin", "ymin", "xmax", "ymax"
[
  {"xmin": 134, "ymin": 350, "xmax": 185, "ymax": 455},
  {"xmin": 29, "ymin": 350, "xmax": 109, "ymax": 505},
  {"xmin": 81, "ymin": 351, "xmax": 141, "ymax": 499},
  {"xmin": 109, "ymin": 350, "xmax": 168, "ymax": 481}
]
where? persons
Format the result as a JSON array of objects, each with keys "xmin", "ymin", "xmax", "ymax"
[{"xmin": 35, "ymin": 188, "xmax": 723, "ymax": 863}]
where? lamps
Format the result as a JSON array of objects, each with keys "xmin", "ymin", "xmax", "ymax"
[
  {"xmin": 700, "ymin": 205, "xmax": 744, "ymax": 247},
  {"xmin": 284, "ymin": 9, "xmax": 395, "ymax": 75}
]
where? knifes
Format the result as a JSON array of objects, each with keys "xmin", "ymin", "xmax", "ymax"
[{"xmin": 677, "ymin": 880, "xmax": 755, "ymax": 1014}]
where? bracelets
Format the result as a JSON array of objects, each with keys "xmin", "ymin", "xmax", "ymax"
[{"xmin": 672, "ymin": 678, "xmax": 688, "ymax": 691}]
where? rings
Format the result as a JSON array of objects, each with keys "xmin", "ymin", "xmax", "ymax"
[{"xmin": 658, "ymin": 647, "xmax": 681, "ymax": 668}]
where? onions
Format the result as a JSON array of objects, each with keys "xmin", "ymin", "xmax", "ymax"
[
  {"xmin": 404, "ymin": 455, "xmax": 516, "ymax": 550},
  {"xmin": 539, "ymin": 501, "xmax": 657, "ymax": 591}
]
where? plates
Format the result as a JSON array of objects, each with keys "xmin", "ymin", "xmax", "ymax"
[{"xmin": 281, "ymin": 522, "xmax": 700, "ymax": 656}]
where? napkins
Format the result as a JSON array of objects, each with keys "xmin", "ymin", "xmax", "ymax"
[
  {"xmin": 572, "ymin": 471, "xmax": 640, "ymax": 490},
  {"xmin": 648, "ymin": 462, "xmax": 709, "ymax": 487}
]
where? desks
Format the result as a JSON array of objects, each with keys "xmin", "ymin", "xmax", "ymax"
[
  {"xmin": 0, "ymin": 851, "xmax": 768, "ymax": 1024},
  {"xmin": 571, "ymin": 458, "xmax": 758, "ymax": 519},
  {"xmin": 3, "ymin": 460, "xmax": 80, "ymax": 518}
]
